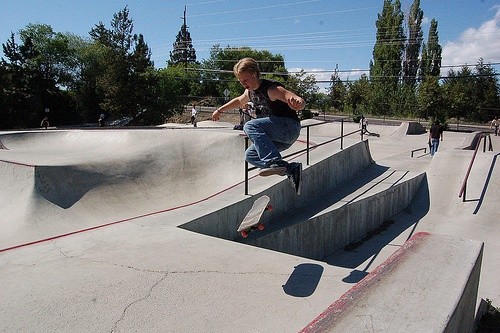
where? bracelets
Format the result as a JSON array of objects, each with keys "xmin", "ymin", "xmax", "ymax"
[{"xmin": 216, "ymin": 108, "xmax": 222, "ymax": 114}]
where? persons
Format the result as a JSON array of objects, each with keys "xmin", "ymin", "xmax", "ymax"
[
  {"xmin": 427, "ymin": 120, "xmax": 444, "ymax": 154},
  {"xmin": 99, "ymin": 113, "xmax": 105, "ymax": 127},
  {"xmin": 191, "ymin": 102, "xmax": 197, "ymax": 126},
  {"xmin": 40, "ymin": 116, "xmax": 50, "ymax": 129},
  {"xmin": 490, "ymin": 116, "xmax": 500, "ymax": 136},
  {"xmin": 359, "ymin": 116, "xmax": 368, "ymax": 134},
  {"xmin": 212, "ymin": 57, "xmax": 306, "ymax": 196}
]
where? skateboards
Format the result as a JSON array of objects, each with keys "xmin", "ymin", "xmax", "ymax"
[
  {"xmin": 236, "ymin": 195, "xmax": 272, "ymax": 238},
  {"xmin": 369, "ymin": 133, "xmax": 380, "ymax": 137},
  {"xmin": 497, "ymin": 125, "xmax": 500, "ymax": 133},
  {"xmin": 427, "ymin": 137, "xmax": 431, "ymax": 154},
  {"xmin": 191, "ymin": 115, "xmax": 195, "ymax": 124}
]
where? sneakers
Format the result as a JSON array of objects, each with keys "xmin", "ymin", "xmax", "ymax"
[
  {"xmin": 258, "ymin": 160, "xmax": 288, "ymax": 176},
  {"xmin": 288, "ymin": 162, "xmax": 302, "ymax": 195}
]
